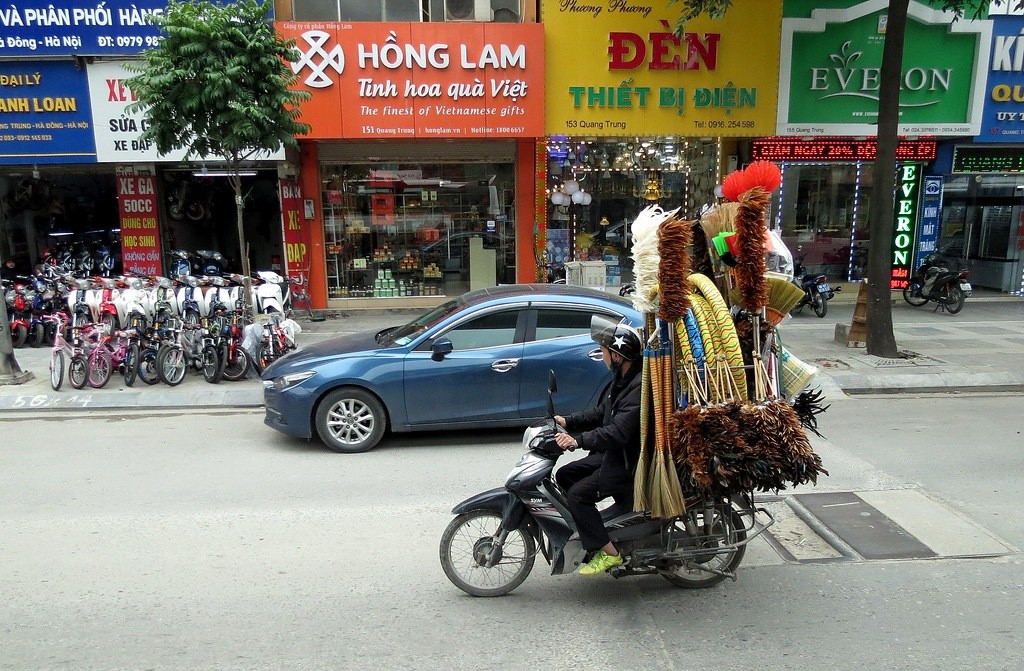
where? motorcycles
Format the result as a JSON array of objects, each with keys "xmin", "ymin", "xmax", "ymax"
[
  {"xmin": 793, "ymin": 252, "xmax": 834, "ymax": 317},
  {"xmin": 903, "ymin": 247, "xmax": 972, "ymax": 314},
  {"xmin": 439, "ymin": 368, "xmax": 776, "ymax": 597}
]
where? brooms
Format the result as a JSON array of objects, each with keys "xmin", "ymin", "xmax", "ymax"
[
  {"xmin": 699, "ymin": 202, "xmax": 806, "ymax": 356},
  {"xmin": 632, "ymin": 340, "xmax": 686, "ymax": 519}
]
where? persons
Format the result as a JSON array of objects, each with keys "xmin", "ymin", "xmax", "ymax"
[
  {"xmin": 0, "ymin": 254, "xmax": 30, "ymax": 291},
  {"xmin": 553, "ymin": 323, "xmax": 655, "ymax": 575}
]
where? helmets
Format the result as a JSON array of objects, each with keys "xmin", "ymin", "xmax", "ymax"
[{"xmin": 592, "ymin": 324, "xmax": 642, "ymax": 361}]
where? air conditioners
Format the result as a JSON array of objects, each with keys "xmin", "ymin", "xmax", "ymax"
[{"xmin": 443, "ymin": 0, "xmax": 491, "ymax": 23}]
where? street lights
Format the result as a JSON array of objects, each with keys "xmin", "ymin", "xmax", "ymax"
[{"xmin": 551, "ymin": 180, "xmax": 592, "ymax": 262}]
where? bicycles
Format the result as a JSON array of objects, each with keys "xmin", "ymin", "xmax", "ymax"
[{"xmin": 42, "ymin": 301, "xmax": 298, "ymax": 391}]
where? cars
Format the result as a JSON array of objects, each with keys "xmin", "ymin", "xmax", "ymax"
[
  {"xmin": 398, "ymin": 231, "xmax": 514, "ymax": 272},
  {"xmin": 588, "ymin": 220, "xmax": 634, "ymax": 244},
  {"xmin": 261, "ymin": 284, "xmax": 645, "ymax": 453}
]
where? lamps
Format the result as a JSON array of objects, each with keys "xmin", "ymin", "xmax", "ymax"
[
  {"xmin": 600, "ymin": 215, "xmax": 609, "ymax": 226},
  {"xmin": 548, "ymin": 137, "xmax": 724, "ymax": 208},
  {"xmin": 551, "ymin": 180, "xmax": 592, "ymax": 261},
  {"xmin": 33, "ymin": 166, "xmax": 40, "ymax": 178},
  {"xmin": 191, "ymin": 170, "xmax": 259, "ymax": 177}
]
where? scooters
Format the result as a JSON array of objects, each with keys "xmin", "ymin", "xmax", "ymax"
[{"xmin": 0, "ymin": 239, "xmax": 291, "ymax": 347}]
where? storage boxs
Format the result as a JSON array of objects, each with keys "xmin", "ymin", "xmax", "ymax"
[{"xmin": 565, "ymin": 255, "xmax": 622, "ymax": 289}]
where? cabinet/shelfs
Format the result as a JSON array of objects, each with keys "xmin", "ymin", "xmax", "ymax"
[
  {"xmin": 941, "ymin": 200, "xmax": 1024, "ymax": 294},
  {"xmin": 324, "ymin": 190, "xmax": 515, "ymax": 297}
]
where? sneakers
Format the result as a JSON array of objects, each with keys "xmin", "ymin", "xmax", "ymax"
[{"xmin": 579, "ymin": 551, "xmax": 623, "ymax": 575}]
